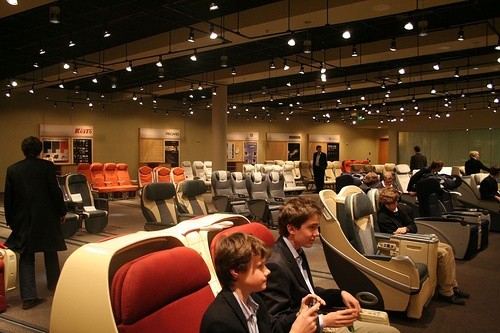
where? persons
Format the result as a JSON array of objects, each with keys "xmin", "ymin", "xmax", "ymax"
[
  {"xmin": 410, "ymin": 145, "xmax": 427, "ymax": 171},
  {"xmin": 465, "ymin": 150, "xmax": 490, "ymax": 175},
  {"xmin": 4, "ymin": 136, "xmax": 67, "ymax": 308},
  {"xmin": 479, "ymin": 165, "xmax": 500, "ymax": 202},
  {"xmin": 407, "ymin": 159, "xmax": 444, "ymax": 195},
  {"xmin": 313, "ymin": 145, "xmax": 328, "ymax": 194},
  {"xmin": 256, "ymin": 196, "xmax": 362, "ymax": 332},
  {"xmin": 377, "ymin": 172, "xmax": 399, "ymax": 189},
  {"xmin": 200, "ymin": 231, "xmax": 326, "ymax": 332},
  {"xmin": 378, "ymin": 186, "xmax": 470, "ymax": 305},
  {"xmin": 359, "ymin": 171, "xmax": 378, "ymax": 194}
]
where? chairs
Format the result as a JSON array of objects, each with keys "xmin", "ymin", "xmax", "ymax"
[
  {"xmin": 193, "ymin": 161, "xmax": 211, "ymax": 186},
  {"xmin": 283, "ymin": 161, "xmax": 313, "ymax": 196},
  {"xmin": 172, "ymin": 167, "xmax": 188, "ymax": 188},
  {"xmin": 153, "ymin": 167, "xmax": 164, "ymax": 184},
  {"xmin": 55, "ymin": 176, "xmax": 81, "ymax": 232},
  {"xmin": 412, "ymin": 215, "xmax": 489, "ymax": 261},
  {"xmin": 324, "ymin": 159, "xmax": 395, "ymax": 191},
  {"xmin": 177, "ymin": 180, "xmax": 217, "ymax": 218},
  {"xmin": 182, "ymin": 161, "xmax": 193, "ymax": 181},
  {"xmin": 138, "ymin": 167, "xmax": 154, "ymax": 197},
  {"xmin": 0, "ymin": 241, "xmax": 16, "ymax": 314},
  {"xmin": 77, "ymin": 163, "xmax": 112, "ymax": 198},
  {"xmin": 66, "ymin": 174, "xmax": 109, "ymax": 235},
  {"xmin": 428, "ymin": 189, "xmax": 490, "ymax": 235},
  {"xmin": 141, "ymin": 183, "xmax": 196, "ymax": 231},
  {"xmin": 157, "ymin": 167, "xmax": 171, "ymax": 183},
  {"xmin": 394, "ymin": 163, "xmax": 412, "ymax": 194},
  {"xmin": 91, "ymin": 162, "xmax": 113, "ymax": 200},
  {"xmin": 159, "ymin": 214, "xmax": 389, "ymax": 333},
  {"xmin": 204, "ymin": 161, "xmax": 212, "ymax": 181},
  {"xmin": 453, "ymin": 173, "xmax": 500, "ymax": 233},
  {"xmin": 49, "ymin": 232, "xmax": 217, "ymax": 333},
  {"xmin": 211, "ymin": 160, "xmax": 283, "ymax": 229},
  {"xmin": 314, "ymin": 189, "xmax": 431, "ymax": 321},
  {"xmin": 116, "ymin": 162, "xmax": 139, "ymax": 199},
  {"xmin": 337, "ymin": 186, "xmax": 455, "ymax": 292},
  {"xmin": 104, "ymin": 162, "xmax": 127, "ymax": 201}
]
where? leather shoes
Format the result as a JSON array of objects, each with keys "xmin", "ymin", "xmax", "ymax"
[
  {"xmin": 22, "ymin": 297, "xmax": 46, "ymax": 309},
  {"xmin": 453, "ymin": 287, "xmax": 470, "ymax": 298},
  {"xmin": 438, "ymin": 292, "xmax": 465, "ymax": 305}
]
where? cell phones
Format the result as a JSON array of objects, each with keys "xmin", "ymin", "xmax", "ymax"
[{"xmin": 310, "ymin": 298, "xmax": 316, "ymax": 308}]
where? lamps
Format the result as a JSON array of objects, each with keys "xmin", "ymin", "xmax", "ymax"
[
  {"xmin": 49, "ymin": 6, "xmax": 61, "ymax": 24},
  {"xmin": 209, "ymin": 24, "xmax": 217, "ymax": 40},
  {"xmin": 6, "ymin": 48, "xmax": 217, "ymax": 116},
  {"xmin": 303, "ymin": 32, "xmax": 312, "ymax": 53},
  {"xmin": 389, "ymin": 36, "xmax": 397, "ymax": 52},
  {"xmin": 457, "ymin": 26, "xmax": 465, "ymax": 42},
  {"xmin": 351, "ymin": 44, "xmax": 359, "ymax": 58},
  {"xmin": 104, "ymin": 29, "xmax": 110, "ymax": 37},
  {"xmin": 288, "ymin": 31, "xmax": 296, "ymax": 46},
  {"xmin": 188, "ymin": 29, "xmax": 194, "ymax": 43},
  {"xmin": 68, "ymin": 38, "xmax": 78, "ymax": 47},
  {"xmin": 405, "ymin": 18, "xmax": 413, "ymax": 30},
  {"xmin": 341, "ymin": 25, "xmax": 351, "ymax": 39},
  {"xmin": 495, "ymin": 37, "xmax": 500, "ymax": 50},
  {"xmin": 209, "ymin": 2, "xmax": 219, "ymax": 11},
  {"xmin": 227, "ymin": 58, "xmax": 500, "ymax": 127}
]
